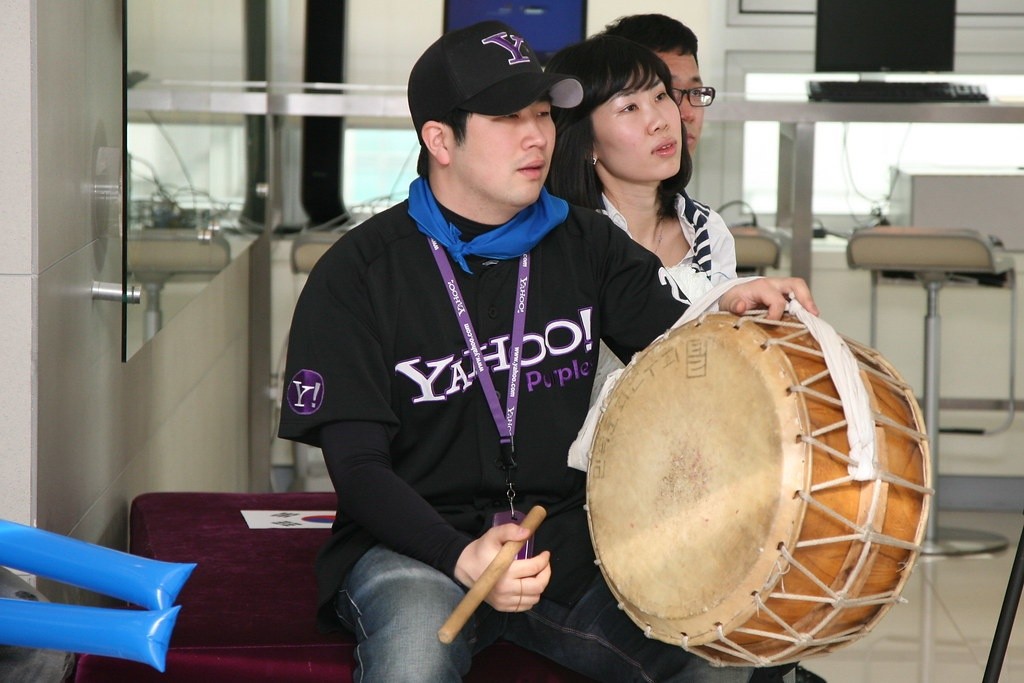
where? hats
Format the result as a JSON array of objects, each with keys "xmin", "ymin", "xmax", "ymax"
[{"xmin": 407, "ymin": 20, "xmax": 588, "ymax": 145}]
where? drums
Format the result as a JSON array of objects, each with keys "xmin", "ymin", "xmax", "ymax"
[{"xmin": 584, "ymin": 302, "xmax": 933, "ymax": 672}]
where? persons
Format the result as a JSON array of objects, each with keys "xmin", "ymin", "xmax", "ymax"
[
  {"xmin": 278, "ymin": 20, "xmax": 817, "ymax": 683},
  {"xmin": 542, "ymin": 13, "xmax": 740, "ymax": 413}
]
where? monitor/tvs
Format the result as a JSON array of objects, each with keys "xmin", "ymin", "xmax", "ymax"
[
  {"xmin": 814, "ymin": 0, "xmax": 956, "ymax": 74},
  {"xmin": 443, "ymin": 0, "xmax": 588, "ymax": 66}
]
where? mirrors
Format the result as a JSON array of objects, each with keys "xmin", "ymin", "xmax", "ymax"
[{"xmin": 122, "ymin": 1, "xmax": 270, "ymax": 361}]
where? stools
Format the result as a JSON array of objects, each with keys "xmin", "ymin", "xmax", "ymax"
[
  {"xmin": 292, "ymin": 231, "xmax": 346, "ymax": 489},
  {"xmin": 848, "ymin": 224, "xmax": 1017, "ymax": 554},
  {"xmin": 127, "ymin": 229, "xmax": 232, "ymax": 343},
  {"xmin": 731, "ymin": 225, "xmax": 782, "ymax": 277}
]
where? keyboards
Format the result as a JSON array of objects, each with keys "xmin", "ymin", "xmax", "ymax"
[{"xmin": 806, "ymin": 80, "xmax": 989, "ymax": 102}]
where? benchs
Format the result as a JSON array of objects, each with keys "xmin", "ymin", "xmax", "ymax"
[{"xmin": 73, "ymin": 493, "xmax": 594, "ymax": 683}]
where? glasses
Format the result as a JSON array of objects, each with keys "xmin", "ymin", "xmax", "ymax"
[{"xmin": 672, "ymin": 87, "xmax": 716, "ymax": 108}]
